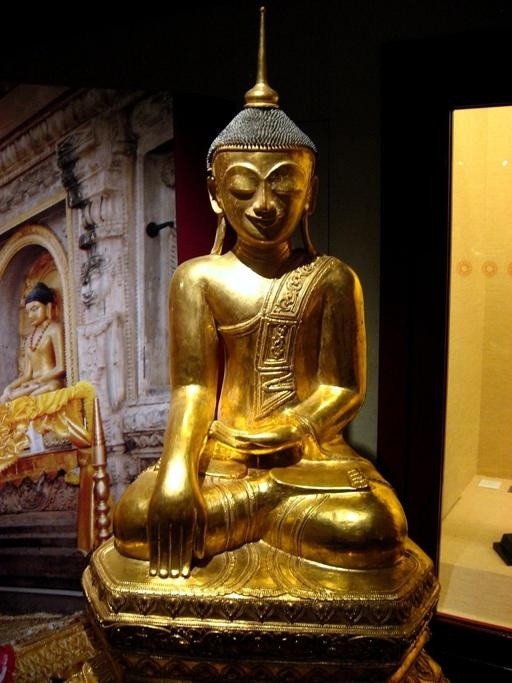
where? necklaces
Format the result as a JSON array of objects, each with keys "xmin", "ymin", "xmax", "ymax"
[{"xmin": 29, "ymin": 321, "xmax": 51, "ymax": 352}]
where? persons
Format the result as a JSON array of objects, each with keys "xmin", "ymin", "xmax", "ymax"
[
  {"xmin": 112, "ymin": 5, "xmax": 410, "ymax": 580},
  {"xmin": 1, "ymin": 281, "xmax": 64, "ymax": 404}
]
